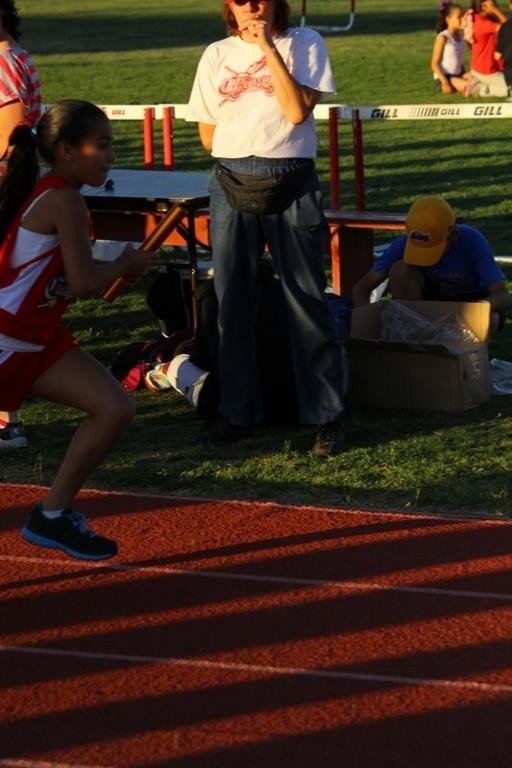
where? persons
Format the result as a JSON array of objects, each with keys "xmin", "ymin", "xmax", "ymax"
[
  {"xmin": 429, "ymin": 2, "xmax": 465, "ymax": 95},
  {"xmin": 2, "ymin": 1, "xmax": 40, "ymax": 191},
  {"xmin": 2, "ymin": 98, "xmax": 136, "ymax": 559},
  {"xmin": 459, "ymin": 0, "xmax": 510, "ymax": 96},
  {"xmin": 191, "ymin": 1, "xmax": 342, "ymax": 458},
  {"xmin": 351, "ymin": 195, "xmax": 511, "ymax": 336}
]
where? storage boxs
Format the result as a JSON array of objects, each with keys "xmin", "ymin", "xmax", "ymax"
[{"xmin": 348, "ymin": 298, "xmax": 491, "ymax": 413}]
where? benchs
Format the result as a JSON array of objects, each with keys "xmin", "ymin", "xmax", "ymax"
[{"xmin": 323, "ymin": 208, "xmax": 407, "ymax": 295}]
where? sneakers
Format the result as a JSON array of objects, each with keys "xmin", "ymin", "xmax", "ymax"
[
  {"xmin": 22, "ymin": 501, "xmax": 117, "ymax": 561},
  {"xmin": 311, "ymin": 427, "xmax": 344, "ymax": 458},
  {"xmin": 211, "ymin": 422, "xmax": 253, "ymax": 443},
  {"xmin": 0, "ymin": 421, "xmax": 28, "ymax": 449}
]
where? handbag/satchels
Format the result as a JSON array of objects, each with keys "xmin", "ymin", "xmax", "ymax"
[{"xmin": 214, "ymin": 162, "xmax": 311, "ymax": 215}]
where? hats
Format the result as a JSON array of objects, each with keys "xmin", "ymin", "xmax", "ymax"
[{"xmin": 403, "ymin": 196, "xmax": 456, "ymax": 267}]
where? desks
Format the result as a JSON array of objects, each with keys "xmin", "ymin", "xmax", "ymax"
[{"xmin": 35, "ymin": 166, "xmax": 211, "ymax": 359}]
